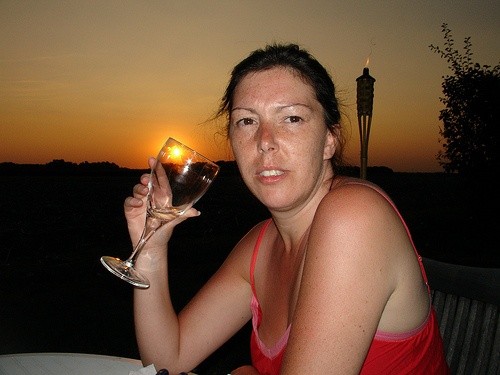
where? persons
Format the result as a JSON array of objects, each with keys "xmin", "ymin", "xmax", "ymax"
[{"xmin": 124, "ymin": 41, "xmax": 444, "ymax": 375}]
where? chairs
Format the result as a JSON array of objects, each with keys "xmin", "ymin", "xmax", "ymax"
[{"xmin": 426, "ymin": 270, "xmax": 500, "ymax": 375}]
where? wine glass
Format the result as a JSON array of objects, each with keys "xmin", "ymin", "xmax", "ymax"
[{"xmin": 100, "ymin": 137, "xmax": 219, "ymax": 288}]
